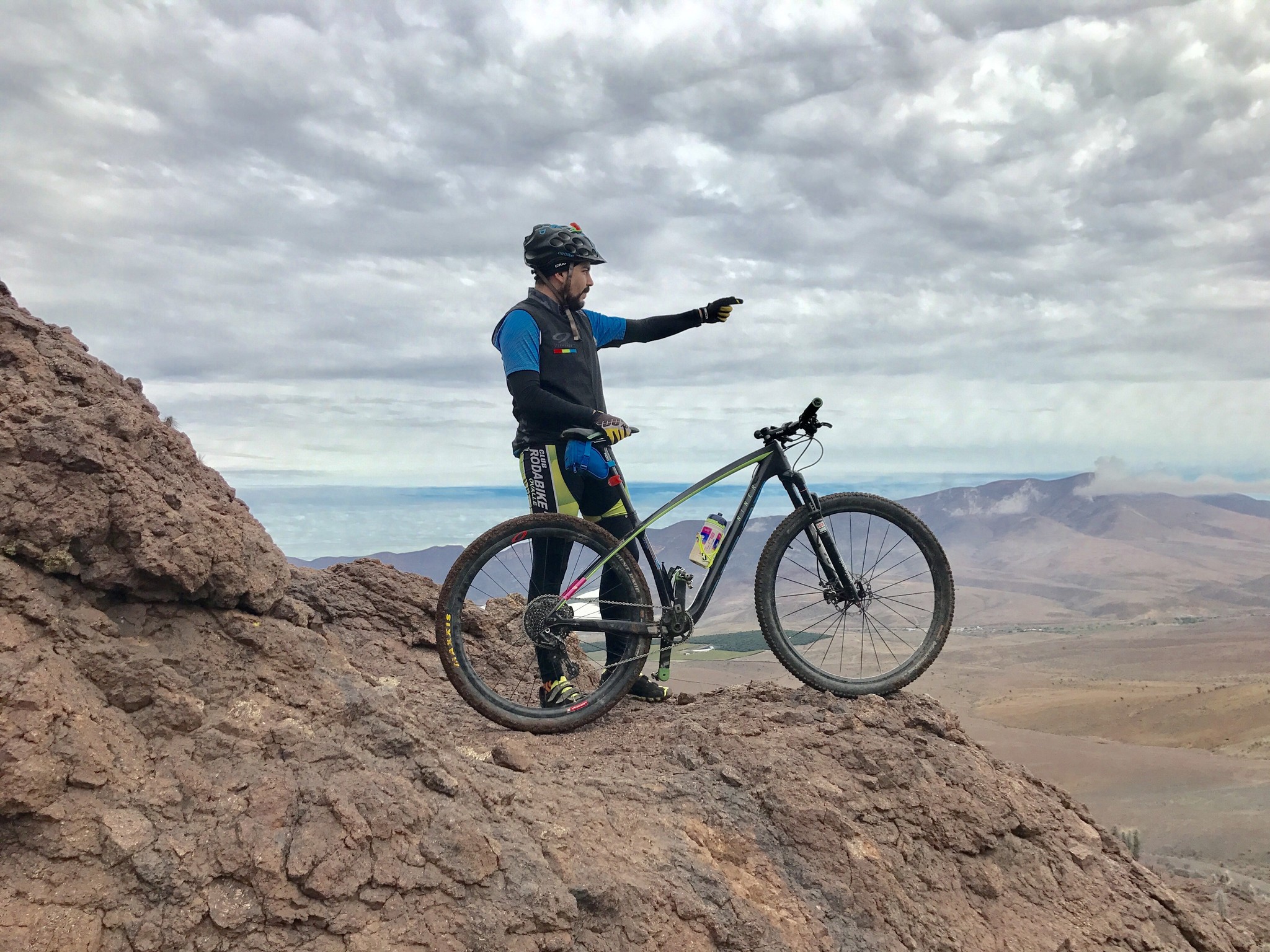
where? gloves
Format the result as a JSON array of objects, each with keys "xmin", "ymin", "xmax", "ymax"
[
  {"xmin": 588, "ymin": 410, "xmax": 632, "ymax": 445},
  {"xmin": 699, "ymin": 296, "xmax": 744, "ymax": 324}
]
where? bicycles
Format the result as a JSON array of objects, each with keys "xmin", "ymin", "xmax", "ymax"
[{"xmin": 435, "ymin": 396, "xmax": 955, "ymax": 735}]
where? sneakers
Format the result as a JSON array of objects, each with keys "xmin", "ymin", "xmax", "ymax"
[
  {"xmin": 599, "ymin": 669, "xmax": 672, "ymax": 702},
  {"xmin": 539, "ymin": 676, "xmax": 585, "ymax": 708}
]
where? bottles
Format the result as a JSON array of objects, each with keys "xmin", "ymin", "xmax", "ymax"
[{"xmin": 689, "ymin": 513, "xmax": 727, "ymax": 569}]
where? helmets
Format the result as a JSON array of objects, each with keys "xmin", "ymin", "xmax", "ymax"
[{"xmin": 523, "ymin": 222, "xmax": 607, "ymax": 269}]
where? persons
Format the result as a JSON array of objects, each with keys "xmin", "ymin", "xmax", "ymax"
[{"xmin": 491, "ymin": 223, "xmax": 744, "ymax": 709}]
